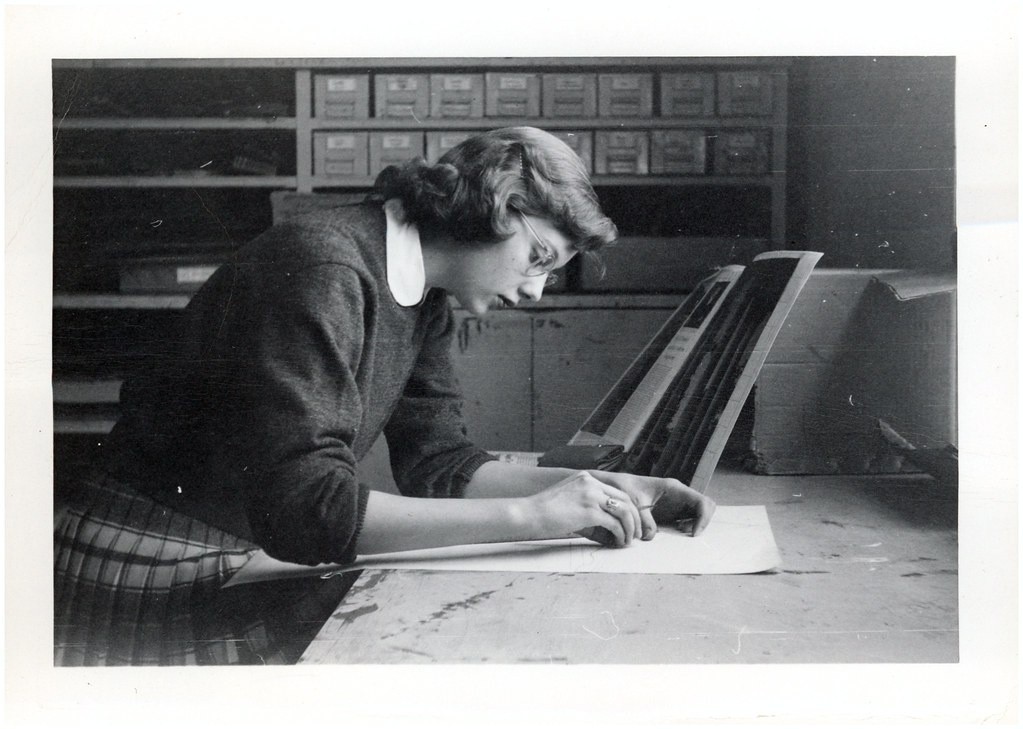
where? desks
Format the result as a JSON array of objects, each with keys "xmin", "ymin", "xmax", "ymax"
[{"xmin": 281, "ymin": 450, "xmax": 960, "ymax": 663}]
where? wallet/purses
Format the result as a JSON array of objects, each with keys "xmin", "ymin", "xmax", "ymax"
[{"xmin": 537, "ymin": 445, "xmax": 625, "ymax": 471}]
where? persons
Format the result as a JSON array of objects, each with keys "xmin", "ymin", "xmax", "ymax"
[{"xmin": 54, "ymin": 127, "xmax": 718, "ymax": 666}]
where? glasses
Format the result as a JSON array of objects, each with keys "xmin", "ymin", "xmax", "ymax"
[{"xmin": 517, "ymin": 210, "xmax": 559, "ymax": 288}]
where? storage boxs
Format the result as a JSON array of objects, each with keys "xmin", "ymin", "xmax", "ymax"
[
  {"xmin": 581, "ymin": 236, "xmax": 747, "ymax": 292},
  {"xmin": 430, "ymin": 73, "xmax": 485, "ymax": 119},
  {"xmin": 313, "ymin": 74, "xmax": 369, "ymax": 118},
  {"xmin": 112, "ymin": 239, "xmax": 241, "ymax": 292},
  {"xmin": 312, "ymin": 132, "xmax": 368, "ymax": 177},
  {"xmin": 650, "ymin": 132, "xmax": 707, "ymax": 175},
  {"xmin": 373, "ymin": 72, "xmax": 429, "ymax": 117},
  {"xmin": 369, "ymin": 130, "xmax": 423, "ymax": 178},
  {"xmin": 660, "ymin": 70, "xmax": 716, "ymax": 120},
  {"xmin": 542, "ymin": 72, "xmax": 596, "ymax": 121},
  {"xmin": 484, "ymin": 74, "xmax": 541, "ymax": 119},
  {"xmin": 426, "ymin": 133, "xmax": 487, "ymax": 169},
  {"xmin": 597, "ymin": 73, "xmax": 655, "ymax": 119},
  {"xmin": 717, "ymin": 71, "xmax": 775, "ymax": 118},
  {"xmin": 551, "ymin": 131, "xmax": 594, "ymax": 174},
  {"xmin": 714, "ymin": 131, "xmax": 769, "ymax": 175},
  {"xmin": 595, "ymin": 132, "xmax": 650, "ymax": 176}
]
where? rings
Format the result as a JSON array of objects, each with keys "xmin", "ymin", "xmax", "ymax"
[{"xmin": 606, "ymin": 497, "xmax": 618, "ymax": 508}]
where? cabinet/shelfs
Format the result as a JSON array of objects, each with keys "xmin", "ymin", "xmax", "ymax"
[{"xmin": 52, "ymin": 59, "xmax": 790, "ymax": 493}]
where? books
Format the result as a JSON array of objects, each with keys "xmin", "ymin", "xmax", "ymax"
[{"xmin": 568, "ymin": 250, "xmax": 825, "ymax": 510}]
